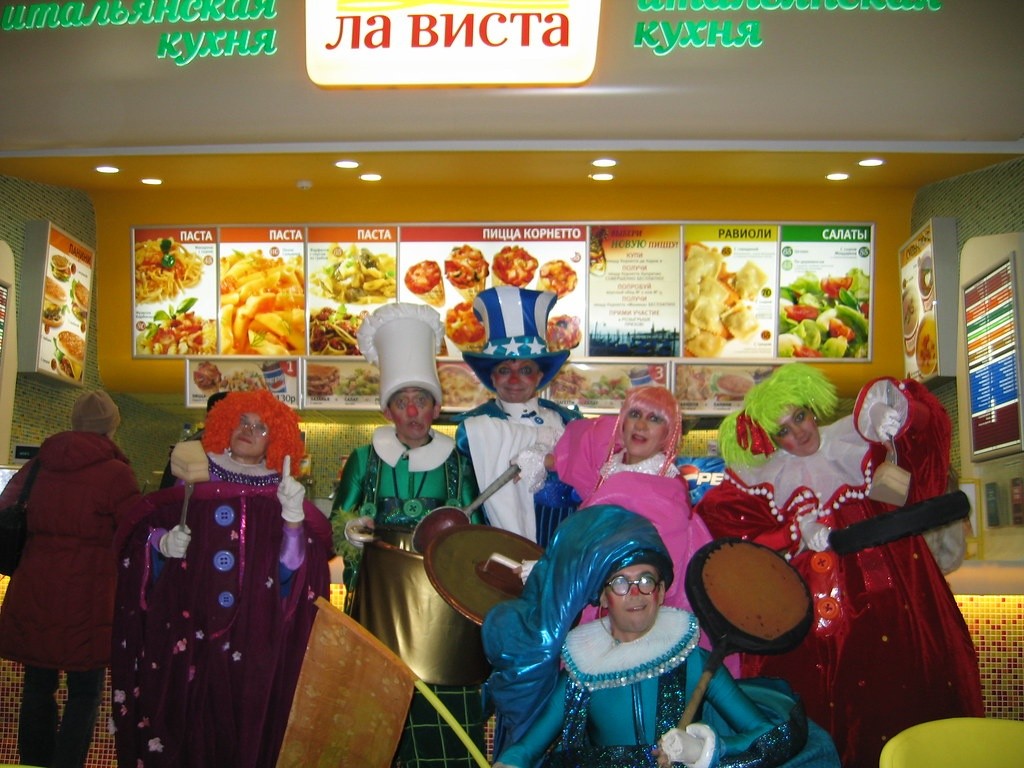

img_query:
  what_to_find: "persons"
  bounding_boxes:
[490,540,841,768]
[0,389,143,768]
[324,368,486,688]
[455,340,588,554]
[577,380,742,679]
[695,358,989,768]
[111,386,333,768]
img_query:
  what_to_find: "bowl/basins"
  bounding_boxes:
[715,369,756,397]
[436,365,477,384]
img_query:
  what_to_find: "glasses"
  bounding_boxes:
[236,420,269,440]
[604,575,660,596]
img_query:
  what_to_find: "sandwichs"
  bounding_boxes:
[306,363,340,397]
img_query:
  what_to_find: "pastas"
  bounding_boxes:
[134,236,207,302]
[136,313,217,357]
[312,241,395,305]
[218,251,307,356]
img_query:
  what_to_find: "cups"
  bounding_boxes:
[902,283,920,357]
[915,311,937,381]
[917,251,934,311]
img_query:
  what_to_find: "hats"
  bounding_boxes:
[71,390,120,434]
[461,286,571,394]
[356,302,446,409]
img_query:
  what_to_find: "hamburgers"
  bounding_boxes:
[41,255,93,383]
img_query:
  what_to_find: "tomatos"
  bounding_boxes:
[819,277,853,294]
[861,303,869,318]
[792,345,821,357]
[829,317,856,341]
[784,305,820,321]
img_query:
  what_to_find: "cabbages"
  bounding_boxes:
[778,269,870,359]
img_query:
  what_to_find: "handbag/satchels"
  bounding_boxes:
[0,458,40,576]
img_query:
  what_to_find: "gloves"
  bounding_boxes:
[869,402,902,444]
[159,525,192,560]
[276,455,305,523]
[798,513,831,552]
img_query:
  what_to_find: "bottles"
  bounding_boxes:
[179,423,194,442]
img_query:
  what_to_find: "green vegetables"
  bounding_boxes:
[580,374,632,400]
[145,296,199,340]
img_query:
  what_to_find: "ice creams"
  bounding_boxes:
[405,245,581,353]
